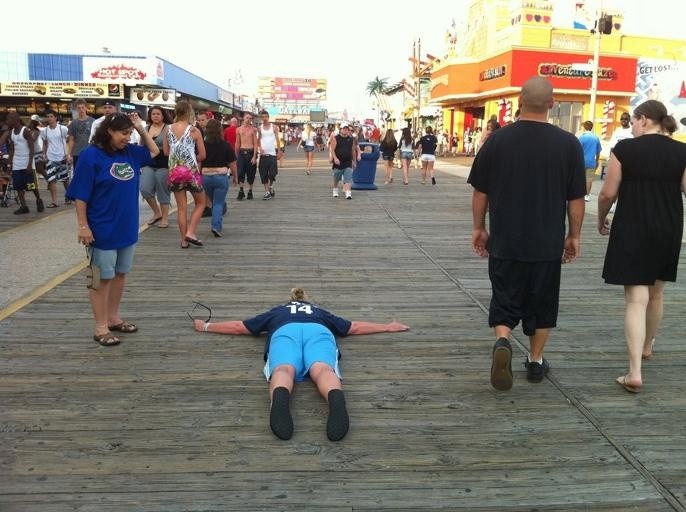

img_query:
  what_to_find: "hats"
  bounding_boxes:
[103,101,115,106]
[340,123,348,128]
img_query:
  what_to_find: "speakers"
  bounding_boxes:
[603,15,612,34]
[599,18,606,32]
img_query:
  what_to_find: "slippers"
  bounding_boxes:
[109,320,138,333]
[643,337,657,360]
[617,375,640,393]
[181,235,203,249]
[94,332,120,346]
[148,216,169,228]
[431,177,436,186]
[46,198,71,208]
[270,387,293,440]
[324,389,349,442]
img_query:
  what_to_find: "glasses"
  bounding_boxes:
[188,300,211,322]
[110,114,128,121]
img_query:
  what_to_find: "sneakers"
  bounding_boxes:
[212,228,222,236]
[489,337,514,390]
[584,195,590,201]
[237,189,252,199]
[346,191,351,199]
[14,199,44,214]
[332,188,338,197]
[525,355,549,383]
[263,185,275,200]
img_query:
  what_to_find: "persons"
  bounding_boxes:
[466,77,587,391]
[437,109,520,158]
[193,286,410,440]
[596,99,686,392]
[63,112,161,346]
[0,100,284,247]
[578,112,635,212]
[284,123,438,199]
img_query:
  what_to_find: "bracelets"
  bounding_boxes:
[203,323,211,331]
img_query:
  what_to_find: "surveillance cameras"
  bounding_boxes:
[590,28,596,34]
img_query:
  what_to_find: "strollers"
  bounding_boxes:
[0,155,22,207]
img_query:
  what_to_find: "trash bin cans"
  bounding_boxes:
[351,142,381,190]
